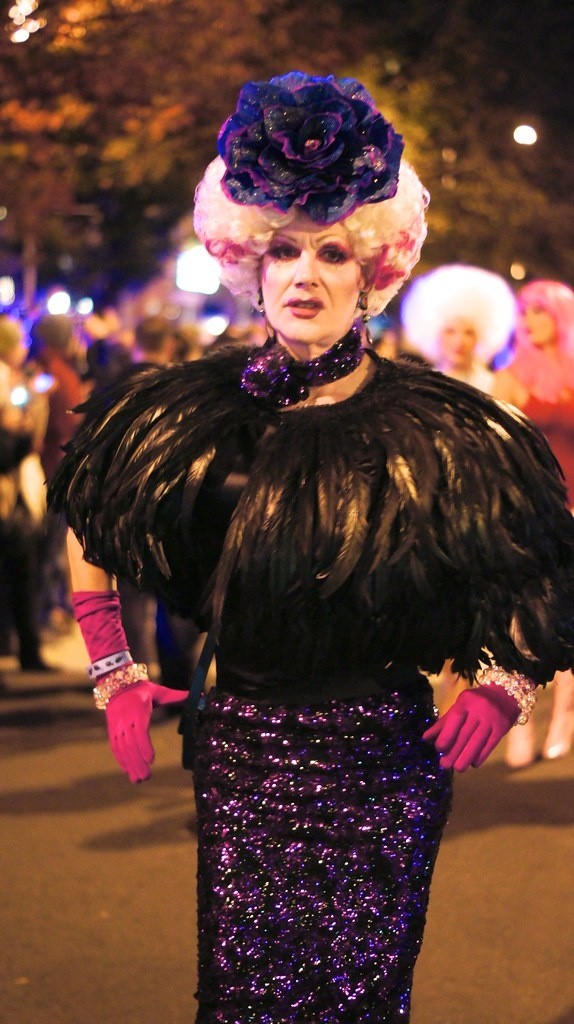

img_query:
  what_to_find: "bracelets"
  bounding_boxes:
[477,663,539,726]
[93,662,148,709]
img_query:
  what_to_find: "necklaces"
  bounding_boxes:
[242,325,363,410]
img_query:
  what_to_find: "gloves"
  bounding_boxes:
[421,681,521,773]
[70,590,190,784]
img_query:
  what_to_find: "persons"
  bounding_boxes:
[0,302,275,711]
[361,264,574,768]
[43,69,574,1024]
